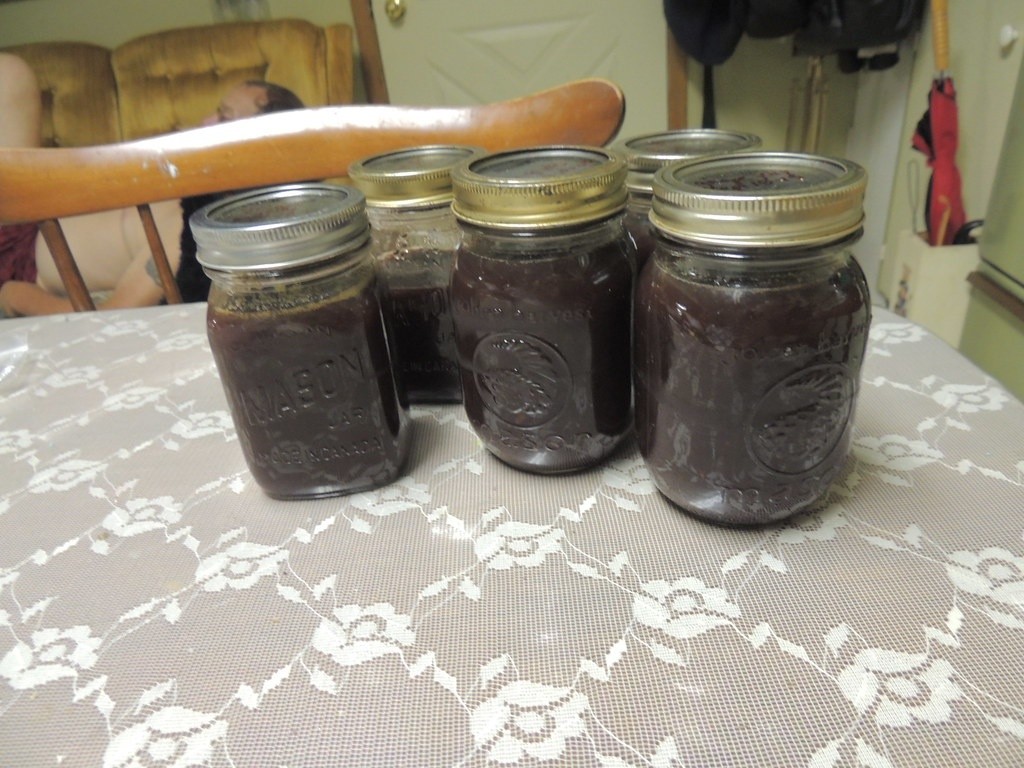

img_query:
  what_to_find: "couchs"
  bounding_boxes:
[0,19,371,187]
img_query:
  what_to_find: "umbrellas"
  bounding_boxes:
[911,0,964,245]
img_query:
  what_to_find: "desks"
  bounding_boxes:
[2,301,1024,768]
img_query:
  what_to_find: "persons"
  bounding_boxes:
[0,51,308,316]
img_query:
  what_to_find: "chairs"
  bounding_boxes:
[0,80,632,313]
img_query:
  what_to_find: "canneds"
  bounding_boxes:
[188,129,873,529]
